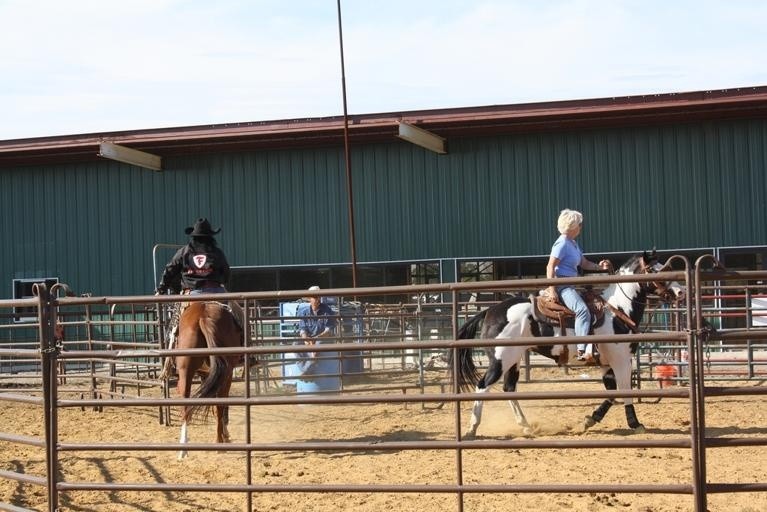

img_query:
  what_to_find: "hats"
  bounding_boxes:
[309,286,320,291]
[183,217,223,236]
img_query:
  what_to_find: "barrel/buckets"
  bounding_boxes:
[292,341,341,393]
[657,365,674,386]
[341,351,364,373]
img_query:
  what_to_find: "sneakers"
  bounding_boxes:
[576,351,600,362]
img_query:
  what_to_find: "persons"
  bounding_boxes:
[291,284,338,383]
[544,208,610,362]
[154,219,262,377]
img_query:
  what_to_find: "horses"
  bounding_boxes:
[445,245,686,441]
[173,300,244,462]
